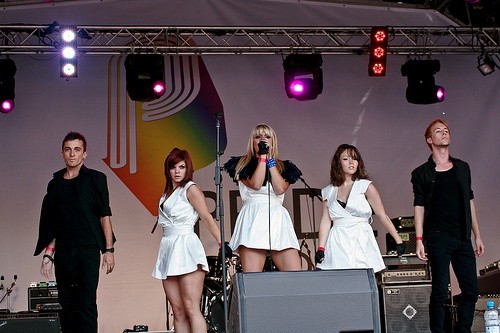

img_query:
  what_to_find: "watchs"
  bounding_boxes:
[106,247,114,252]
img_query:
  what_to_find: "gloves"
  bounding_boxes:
[315,247,325,263]
[396,244,406,256]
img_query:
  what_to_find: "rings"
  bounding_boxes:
[43,254,53,264]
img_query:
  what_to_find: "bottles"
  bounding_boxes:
[483,301,500,333]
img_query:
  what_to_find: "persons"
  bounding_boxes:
[314,143,404,275]
[225,123,302,272]
[152,148,234,333]
[411,119,484,333]
[33,133,117,333]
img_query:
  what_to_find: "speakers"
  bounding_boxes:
[379,283,432,333]
[228,268,381,333]
[0,313,61,333]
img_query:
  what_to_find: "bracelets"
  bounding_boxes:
[267,159,276,168]
[47,247,54,253]
[219,243,221,247]
[258,158,266,163]
[415,237,422,240]
[318,247,324,251]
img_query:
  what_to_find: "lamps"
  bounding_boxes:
[477,51,495,76]
[401,57,446,105]
[282,53,324,101]
[60,26,78,78]
[125,54,166,102]
[369,26,389,78]
[0,58,16,112]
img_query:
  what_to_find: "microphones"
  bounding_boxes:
[265,143,270,149]
[13,275,17,284]
[1,276,4,288]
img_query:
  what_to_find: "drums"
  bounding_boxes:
[208,289,232,333]
[206,255,238,283]
[236,254,271,273]
